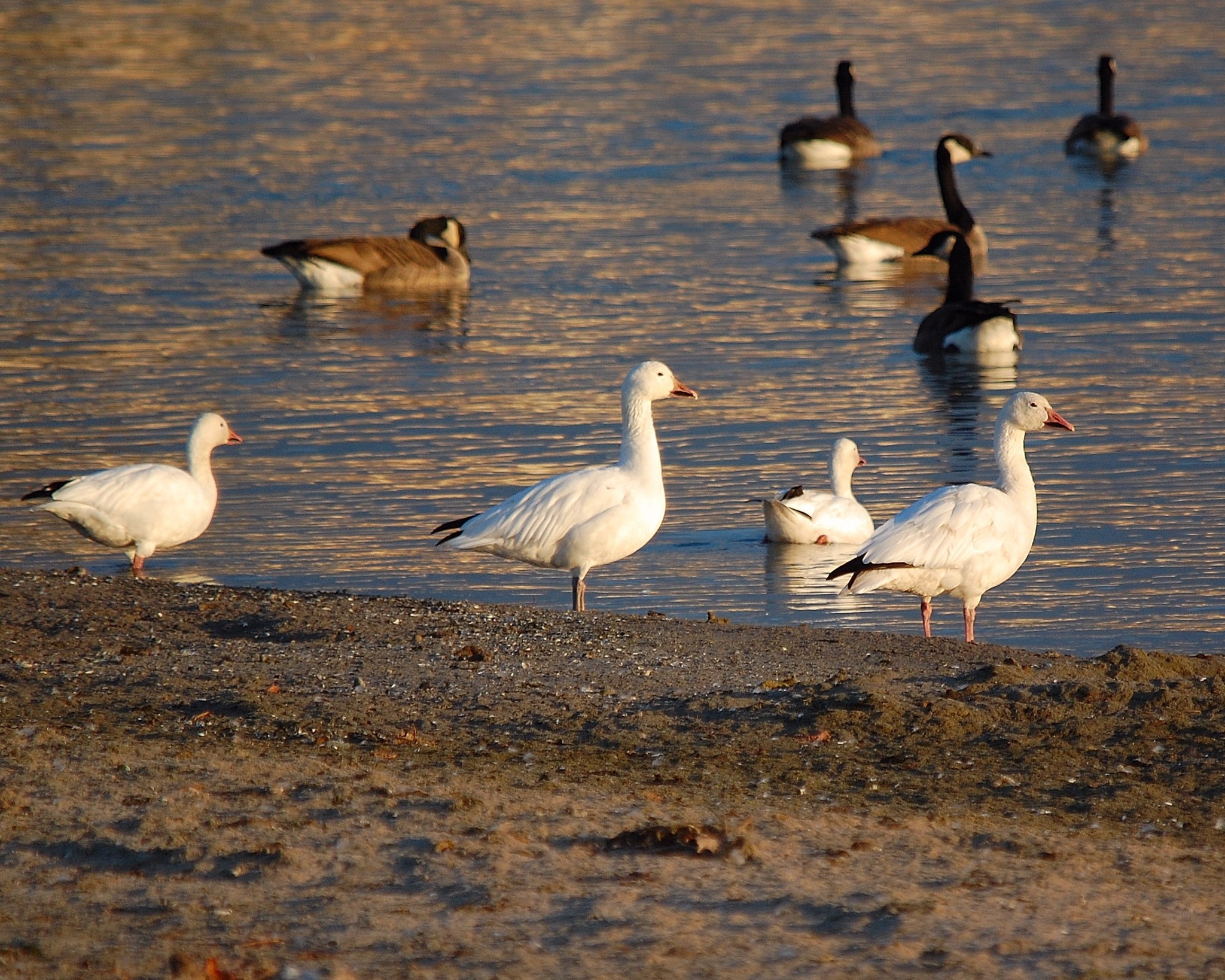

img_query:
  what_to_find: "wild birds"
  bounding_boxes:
[259,216,474,301]
[780,59,1025,364]
[22,411,243,583]
[743,392,1075,644]
[1064,51,1149,163]
[428,359,698,612]
